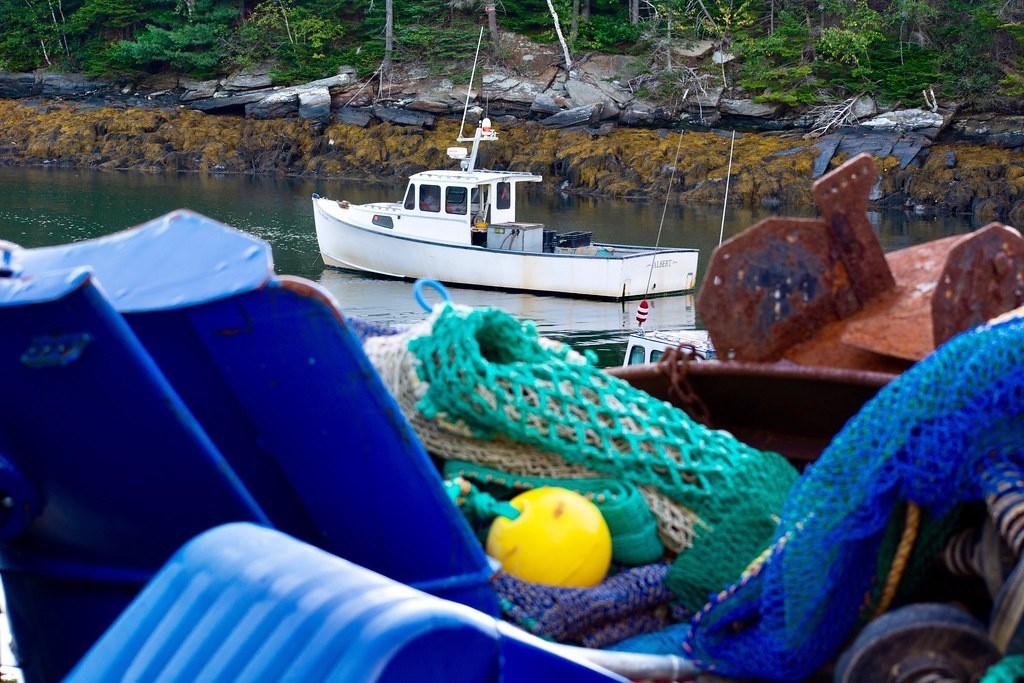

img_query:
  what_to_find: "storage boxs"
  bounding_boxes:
[542,230,593,253]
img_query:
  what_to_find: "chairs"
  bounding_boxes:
[421,202,429,210]
[436,200,452,212]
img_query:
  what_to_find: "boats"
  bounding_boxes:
[310,26,700,301]
[622,126,734,366]
[314,266,699,347]
